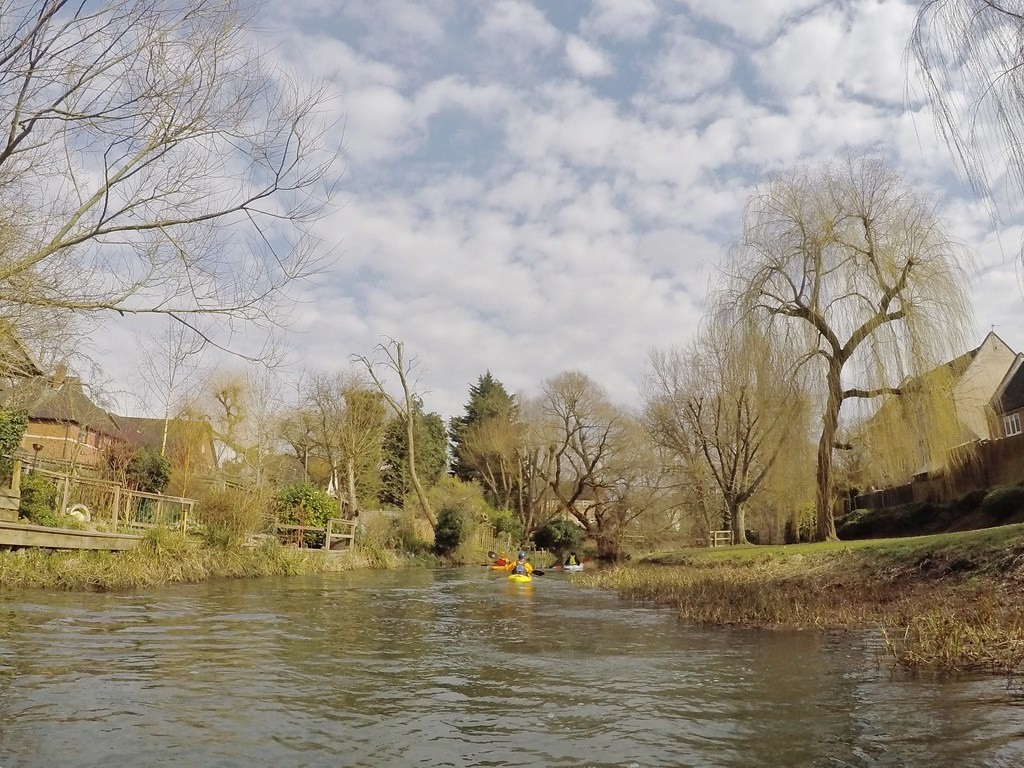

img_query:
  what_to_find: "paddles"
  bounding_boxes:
[488,551,546,576]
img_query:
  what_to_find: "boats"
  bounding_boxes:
[490,565,507,571]
[507,573,532,583]
[563,563,584,573]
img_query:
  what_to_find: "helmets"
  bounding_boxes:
[571,550,576,555]
[518,552,526,557]
[500,551,505,555]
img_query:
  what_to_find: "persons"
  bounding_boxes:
[505,552,533,578]
[565,551,581,566]
